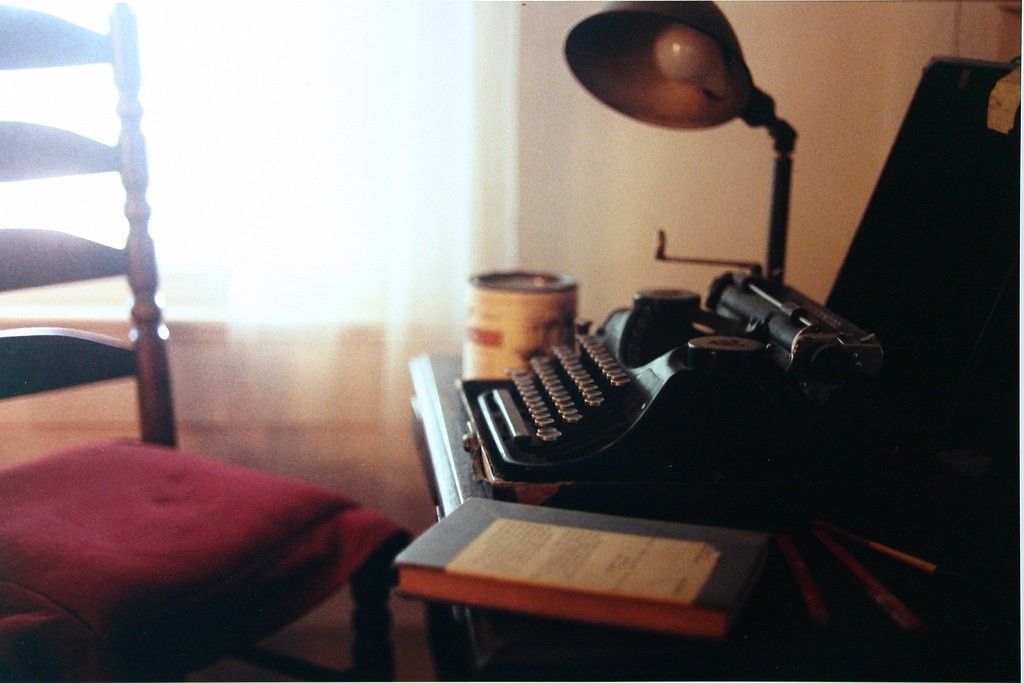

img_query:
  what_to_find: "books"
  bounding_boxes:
[390,495,774,640]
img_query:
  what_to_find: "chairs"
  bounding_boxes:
[0,1,416,682]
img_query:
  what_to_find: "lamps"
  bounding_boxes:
[564,0,802,278]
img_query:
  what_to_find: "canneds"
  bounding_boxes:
[461,270,576,381]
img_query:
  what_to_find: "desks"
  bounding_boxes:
[406,346,1020,682]
